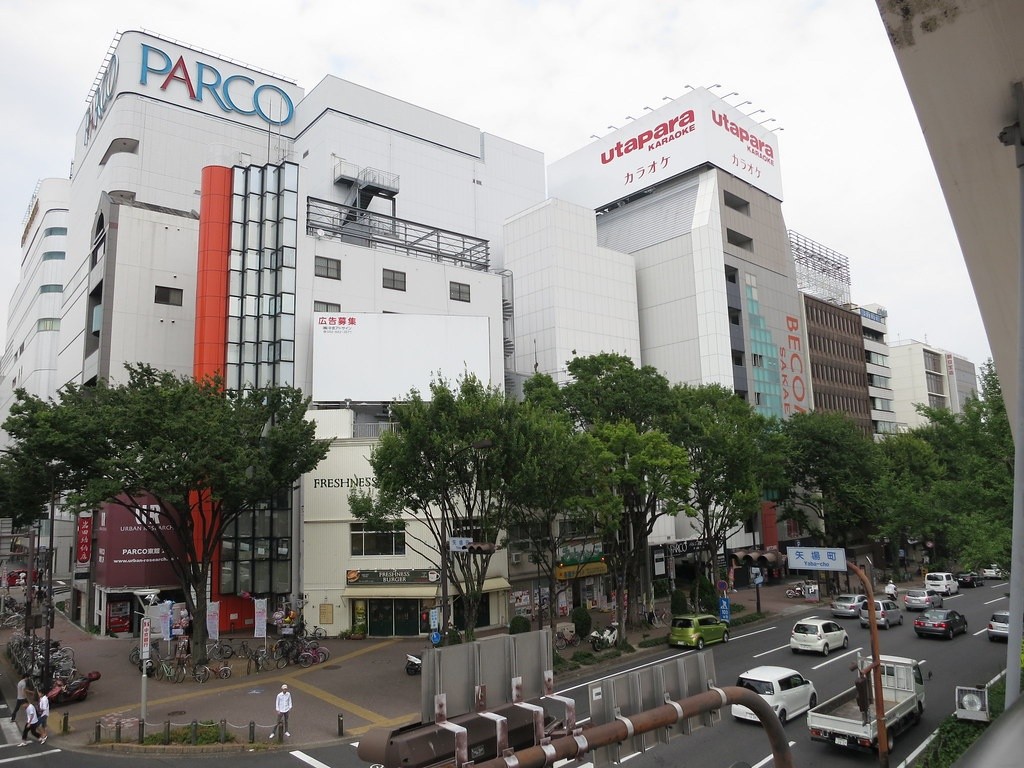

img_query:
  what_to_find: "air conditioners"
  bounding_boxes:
[511,553,522,564]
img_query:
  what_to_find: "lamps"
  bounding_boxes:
[746,109,766,118]
[721,92,739,100]
[707,83,721,91]
[643,106,653,112]
[591,134,601,139]
[661,95,674,102]
[771,126,784,133]
[734,100,752,109]
[625,116,636,121]
[685,84,698,92]
[759,118,776,125]
[608,124,618,130]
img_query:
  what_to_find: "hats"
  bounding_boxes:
[889,580,893,584]
[282,685,288,690]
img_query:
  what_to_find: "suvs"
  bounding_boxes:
[904,588,943,612]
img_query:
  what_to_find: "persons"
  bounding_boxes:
[19,569,48,612]
[10,674,49,747]
[269,684,292,738]
[888,579,898,598]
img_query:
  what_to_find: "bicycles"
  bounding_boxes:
[0,597,83,697]
[650,606,673,629]
[128,620,331,685]
[556,626,580,650]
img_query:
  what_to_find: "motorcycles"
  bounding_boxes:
[590,622,619,652]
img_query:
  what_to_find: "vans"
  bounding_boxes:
[859,600,904,630]
[925,572,959,597]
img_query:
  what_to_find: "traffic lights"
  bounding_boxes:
[462,542,496,555]
[730,550,784,569]
[422,611,430,623]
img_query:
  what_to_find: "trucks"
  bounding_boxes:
[806,654,933,756]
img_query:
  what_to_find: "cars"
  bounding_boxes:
[914,607,967,641]
[954,570,984,588]
[0,568,38,587]
[830,593,868,618]
[988,611,1009,642]
[732,665,817,727]
[982,564,1001,580]
[790,619,849,657]
[668,614,731,651]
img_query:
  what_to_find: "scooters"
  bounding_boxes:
[785,578,814,599]
[403,653,422,676]
[886,583,900,602]
[44,670,101,710]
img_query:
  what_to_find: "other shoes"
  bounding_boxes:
[10,719,17,722]
[17,743,27,747]
[41,736,47,744]
[285,732,290,736]
[269,733,274,738]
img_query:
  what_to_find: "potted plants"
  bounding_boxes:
[351,623,367,640]
